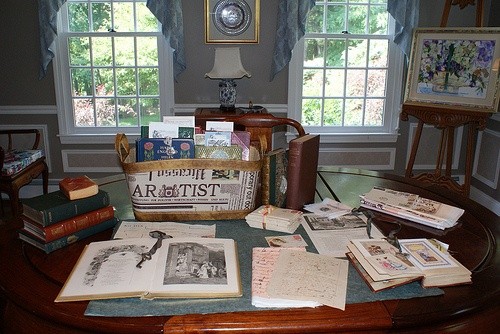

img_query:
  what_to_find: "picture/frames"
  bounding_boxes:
[403,24,500,114]
[203,0,260,46]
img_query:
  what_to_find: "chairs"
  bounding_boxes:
[0,128,49,220]
[219,114,306,153]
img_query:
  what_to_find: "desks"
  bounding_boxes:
[194,106,249,131]
[0,165,500,334]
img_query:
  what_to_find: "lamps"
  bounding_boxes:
[204,47,252,112]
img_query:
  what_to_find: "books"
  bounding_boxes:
[345,238,474,293]
[18,175,116,254]
[135,126,250,161]
[53,238,243,300]
